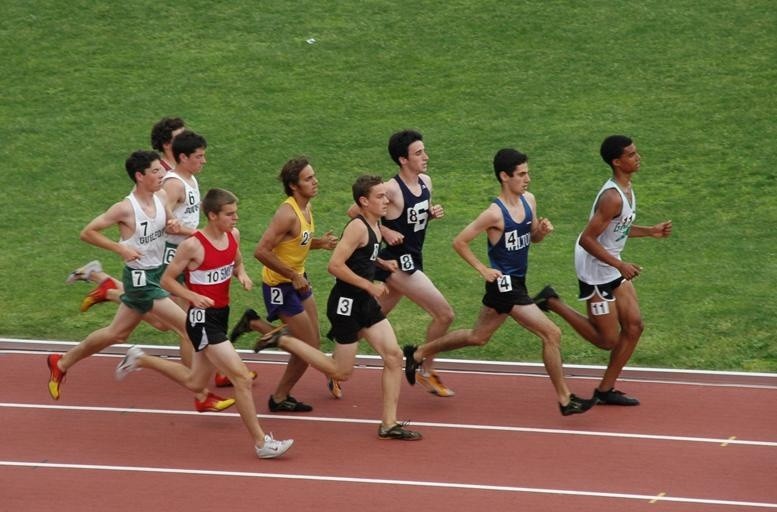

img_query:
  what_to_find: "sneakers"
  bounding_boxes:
[269,392,314,411]
[323,371,343,400]
[404,342,422,384]
[593,388,639,406]
[416,370,455,399]
[229,308,262,343]
[193,392,237,412]
[64,259,102,283]
[114,345,145,381]
[254,322,292,355]
[561,393,592,416]
[254,432,294,458]
[531,284,558,310]
[213,368,257,388]
[376,423,423,441]
[81,276,117,313]
[46,351,66,401]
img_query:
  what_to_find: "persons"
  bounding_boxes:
[254,173,422,440]
[66,117,186,288]
[532,135,672,406]
[115,188,294,459]
[403,148,598,417]
[327,129,454,398]
[80,132,257,387]
[46,150,236,412]
[230,158,337,411]
